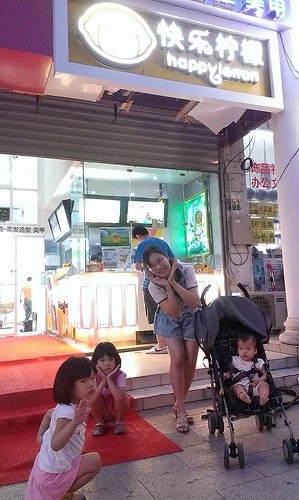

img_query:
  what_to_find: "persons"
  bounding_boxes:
[223,334,269,406]
[132,226,176,354]
[87,341,127,436]
[143,245,203,433]
[25,355,102,500]
[20,276,33,321]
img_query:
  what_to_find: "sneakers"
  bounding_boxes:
[146,344,168,354]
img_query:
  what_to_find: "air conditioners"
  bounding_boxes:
[232,290,287,331]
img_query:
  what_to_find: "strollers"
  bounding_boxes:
[194,283,297,469]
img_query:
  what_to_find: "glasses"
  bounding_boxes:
[149,255,165,271]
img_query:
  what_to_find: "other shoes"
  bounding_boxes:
[114,422,124,434]
[91,424,106,435]
[61,493,86,500]
[176,412,189,432]
[173,405,194,424]
